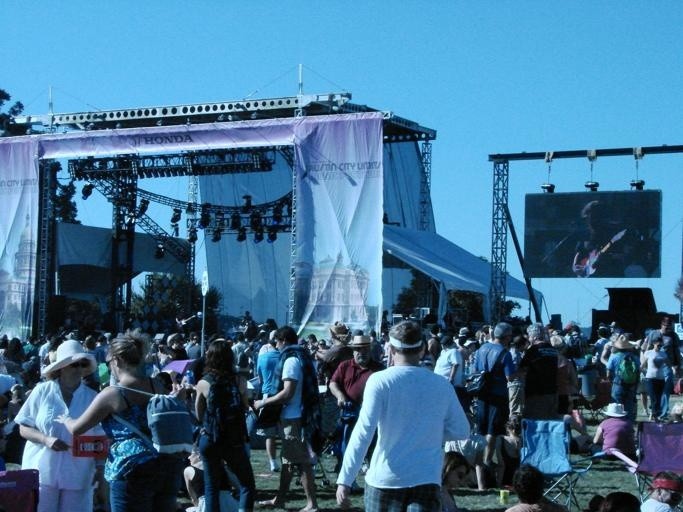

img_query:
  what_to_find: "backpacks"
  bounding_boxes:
[110,384,192,455]
[200,374,251,447]
[615,352,639,385]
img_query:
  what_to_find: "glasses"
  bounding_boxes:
[454,469,466,481]
[70,361,90,369]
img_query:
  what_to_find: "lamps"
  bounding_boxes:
[585,181,599,191]
[541,184,555,192]
[631,180,645,190]
[25,112,294,259]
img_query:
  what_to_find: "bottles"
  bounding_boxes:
[186,368,194,384]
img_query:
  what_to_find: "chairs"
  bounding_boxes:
[520,419,593,512]
[628,422,683,504]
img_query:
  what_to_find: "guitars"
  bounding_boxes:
[572,228,627,278]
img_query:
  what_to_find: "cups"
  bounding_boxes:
[499,489,509,504]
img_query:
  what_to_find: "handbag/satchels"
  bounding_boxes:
[464,370,489,396]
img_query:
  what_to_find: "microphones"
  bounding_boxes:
[571,214,588,227]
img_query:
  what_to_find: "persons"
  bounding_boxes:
[0,309,683,512]
[571,200,636,277]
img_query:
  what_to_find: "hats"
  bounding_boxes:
[43,340,98,378]
[600,403,629,418]
[347,336,378,349]
[608,335,635,350]
[458,327,468,337]
[439,336,451,345]
